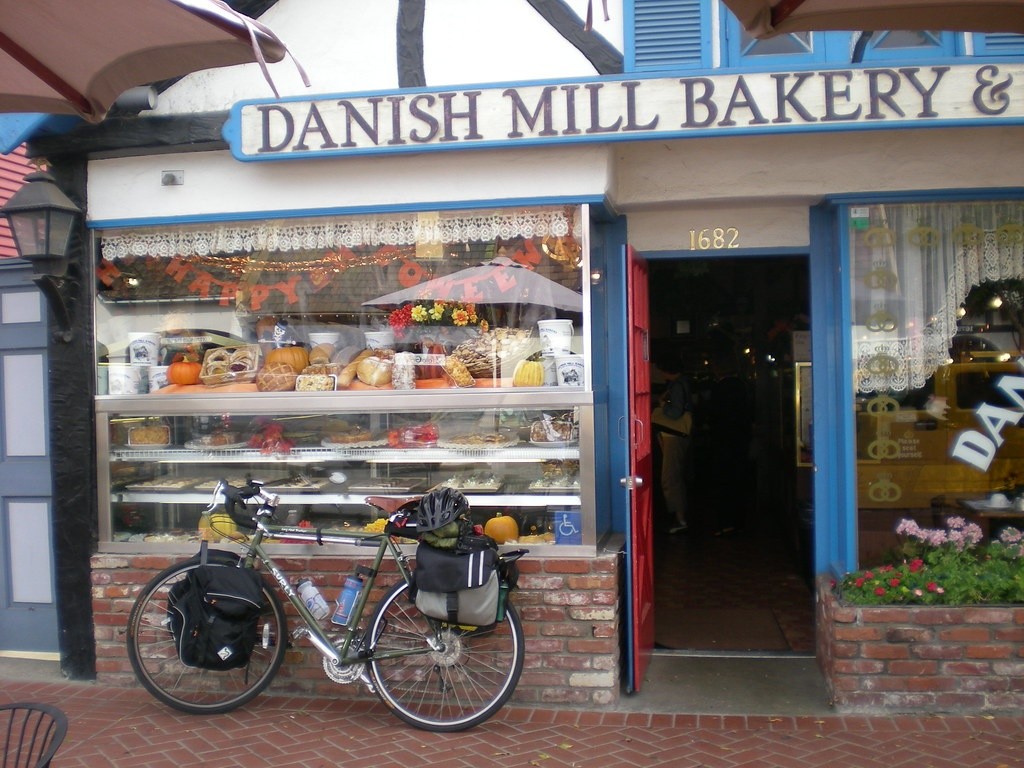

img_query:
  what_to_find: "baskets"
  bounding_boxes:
[199,344,260,387]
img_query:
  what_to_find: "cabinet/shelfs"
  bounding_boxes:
[95,382,612,558]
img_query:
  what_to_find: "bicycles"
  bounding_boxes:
[126,471,525,733]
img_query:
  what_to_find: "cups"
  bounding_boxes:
[363,332,394,350]
[308,332,341,352]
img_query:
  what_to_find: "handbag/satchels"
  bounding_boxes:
[168,564,266,670]
[406,542,499,627]
[650,381,695,438]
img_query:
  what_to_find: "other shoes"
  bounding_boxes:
[666,523,688,534]
[712,528,744,538]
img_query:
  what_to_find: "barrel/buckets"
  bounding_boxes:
[146,366,171,393]
[127,332,160,367]
[535,354,559,386]
[537,318,575,356]
[107,363,142,396]
[554,352,584,386]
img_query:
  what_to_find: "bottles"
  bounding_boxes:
[331,572,362,626]
[295,577,329,620]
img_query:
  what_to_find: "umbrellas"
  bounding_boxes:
[0,0,286,122]
[357,256,585,435]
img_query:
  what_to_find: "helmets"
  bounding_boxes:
[417,487,469,533]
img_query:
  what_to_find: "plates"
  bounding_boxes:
[529,440,580,449]
[124,443,172,449]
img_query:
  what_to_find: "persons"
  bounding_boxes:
[707,350,752,536]
[652,380,695,534]
